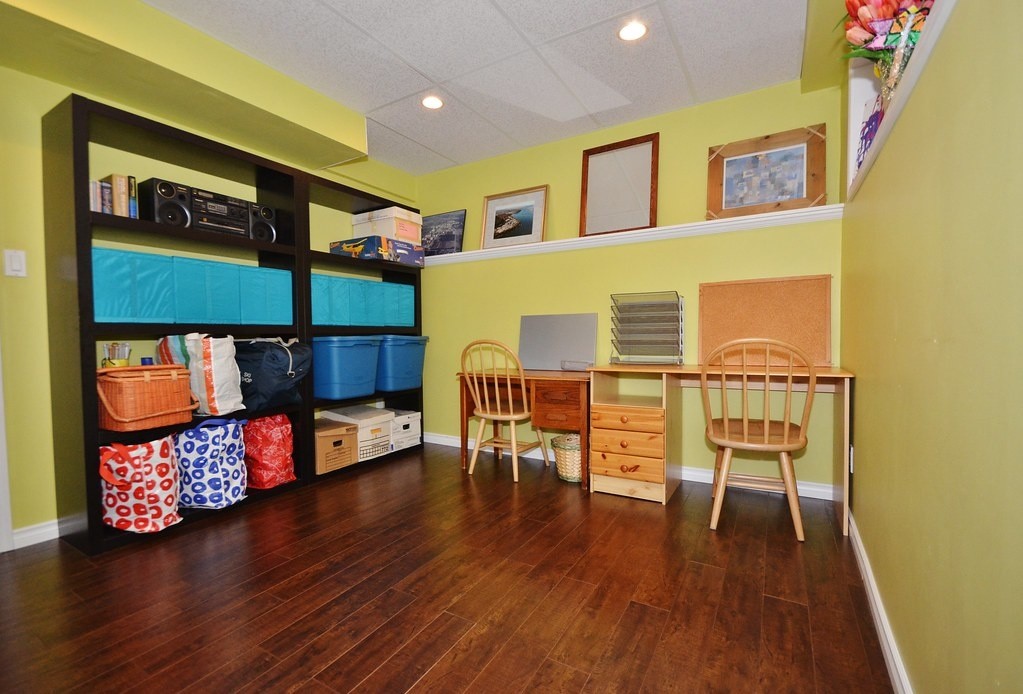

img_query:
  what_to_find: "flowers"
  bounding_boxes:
[832,0,936,67]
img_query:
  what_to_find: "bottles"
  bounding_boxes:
[141,357,153,366]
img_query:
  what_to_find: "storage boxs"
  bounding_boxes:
[321,404,395,463]
[383,282,414,327]
[374,335,430,391]
[383,408,422,453]
[329,235,425,269]
[239,264,293,325]
[172,256,241,324]
[91,246,176,324]
[312,273,350,326]
[312,335,383,401]
[347,277,385,326]
[315,417,358,475]
[351,206,423,246]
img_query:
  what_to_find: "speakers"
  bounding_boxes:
[246,202,276,244]
[138,177,193,228]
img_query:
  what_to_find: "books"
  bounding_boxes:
[88,173,140,220]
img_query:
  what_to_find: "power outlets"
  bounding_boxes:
[849,443,854,474]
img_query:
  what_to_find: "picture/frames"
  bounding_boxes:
[706,122,827,220]
[422,208,467,256]
[479,183,551,249]
[578,132,660,237]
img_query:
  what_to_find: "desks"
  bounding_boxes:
[455,367,591,490]
[587,364,856,536]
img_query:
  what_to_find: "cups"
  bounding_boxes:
[102,358,128,368]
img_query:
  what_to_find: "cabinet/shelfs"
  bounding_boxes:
[41,92,425,557]
[610,291,685,366]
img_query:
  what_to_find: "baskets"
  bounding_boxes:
[551,434,582,483]
[97,364,200,432]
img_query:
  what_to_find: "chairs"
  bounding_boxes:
[460,338,550,483]
[700,337,818,542]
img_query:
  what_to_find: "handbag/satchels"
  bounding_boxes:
[175,419,250,511]
[243,414,298,490]
[233,337,312,413]
[155,333,247,417]
[100,434,185,535]
